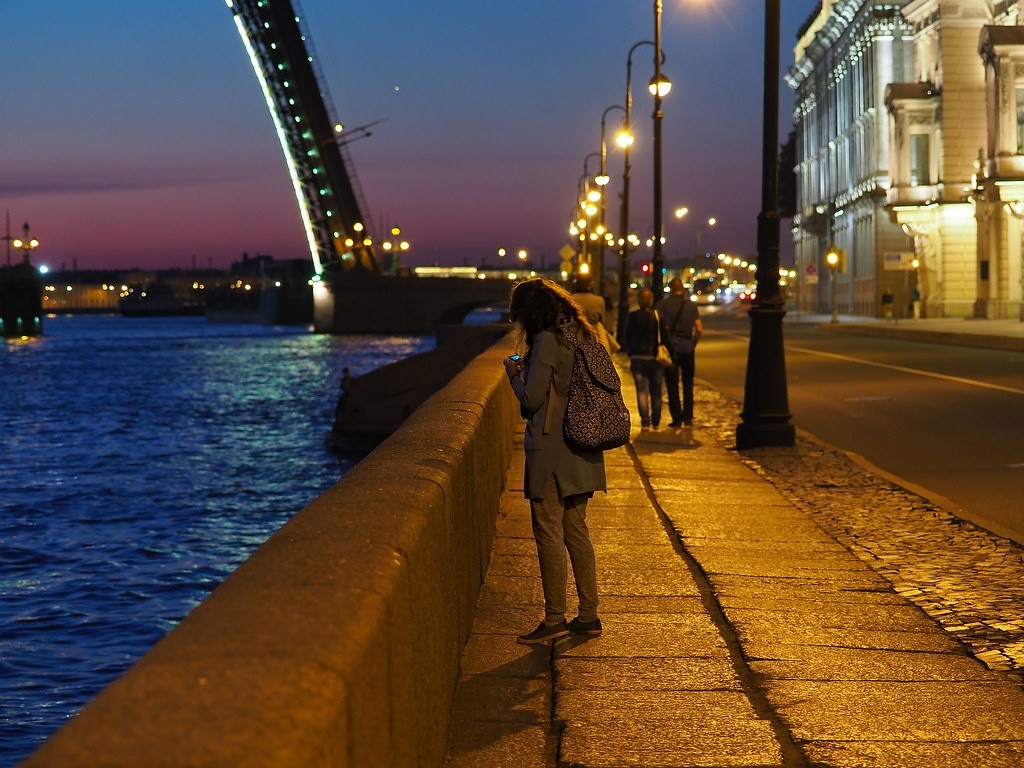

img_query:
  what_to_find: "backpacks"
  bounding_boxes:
[555,320,632,450]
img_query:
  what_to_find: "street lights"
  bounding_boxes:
[597,105,635,309]
[615,41,658,343]
[568,153,610,294]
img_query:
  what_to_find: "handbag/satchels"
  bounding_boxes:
[656,343,671,369]
[660,338,672,353]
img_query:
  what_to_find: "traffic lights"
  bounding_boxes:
[827,253,839,265]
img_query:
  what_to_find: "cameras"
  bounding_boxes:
[508,354,520,363]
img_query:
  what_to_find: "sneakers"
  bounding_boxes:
[567,618,602,635]
[517,620,568,643]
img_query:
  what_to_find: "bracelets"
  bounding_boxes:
[510,374,520,385]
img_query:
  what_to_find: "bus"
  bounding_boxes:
[692,273,717,306]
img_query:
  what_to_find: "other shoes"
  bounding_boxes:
[684,420,693,426]
[652,424,658,429]
[641,426,649,431]
[668,422,681,427]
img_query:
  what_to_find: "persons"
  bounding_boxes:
[661,279,703,429]
[624,288,664,432]
[912,285,921,321]
[569,276,606,325]
[602,297,615,337]
[882,287,894,322]
[503,277,608,644]
